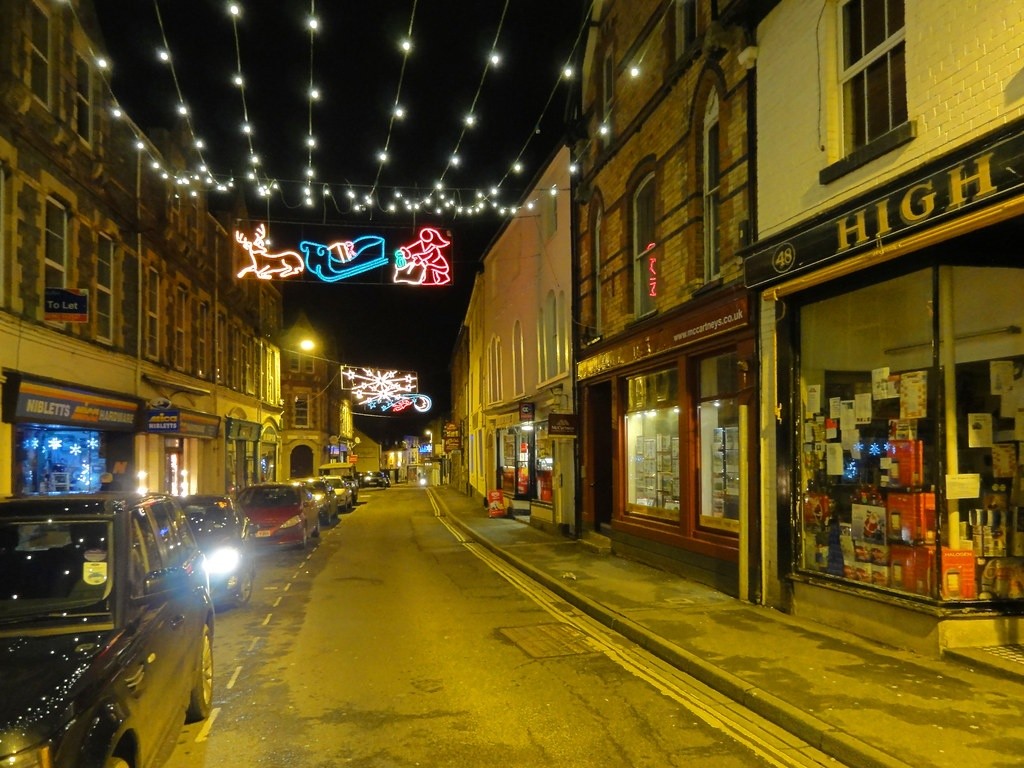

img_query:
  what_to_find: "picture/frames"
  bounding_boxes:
[634,435,680,500]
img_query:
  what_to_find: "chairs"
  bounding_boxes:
[45,524,107,598]
[0,525,24,564]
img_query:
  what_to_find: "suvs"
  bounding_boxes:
[0,490,216,768]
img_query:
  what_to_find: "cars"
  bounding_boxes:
[341,476,359,502]
[325,477,353,511]
[235,482,320,550]
[171,494,256,608]
[290,478,338,526]
[363,471,390,487]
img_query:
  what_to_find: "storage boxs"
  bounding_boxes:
[804,437,1024,601]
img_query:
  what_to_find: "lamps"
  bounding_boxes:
[883,326,1022,355]
